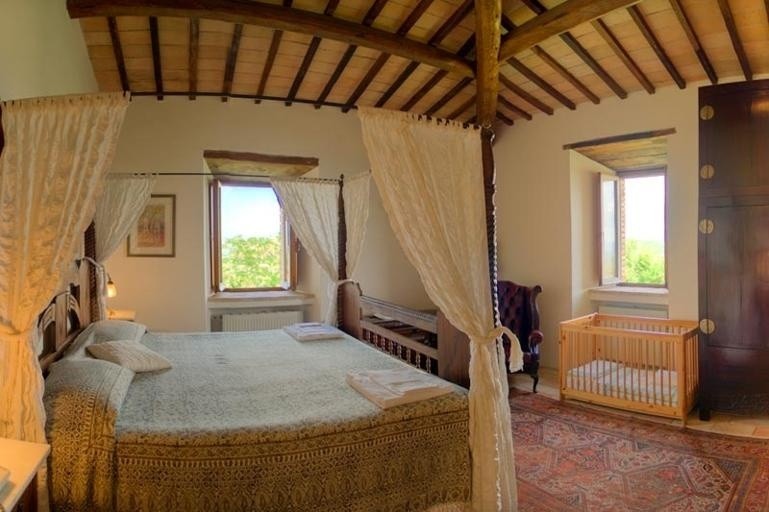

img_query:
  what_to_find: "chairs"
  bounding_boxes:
[496,280,544,392]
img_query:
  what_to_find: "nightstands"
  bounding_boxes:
[0,439,51,512]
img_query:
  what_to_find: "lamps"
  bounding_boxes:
[477,111,497,145]
[75,256,115,298]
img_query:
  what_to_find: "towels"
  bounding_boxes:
[347,366,455,413]
[281,321,343,341]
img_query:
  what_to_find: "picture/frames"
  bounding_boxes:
[127,194,176,258]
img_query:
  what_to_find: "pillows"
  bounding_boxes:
[85,338,174,373]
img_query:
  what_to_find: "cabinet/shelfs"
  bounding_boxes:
[697,86,769,423]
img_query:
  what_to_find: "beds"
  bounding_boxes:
[560,311,699,426]
[39,279,474,512]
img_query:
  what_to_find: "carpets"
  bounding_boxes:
[507,386,768,512]
